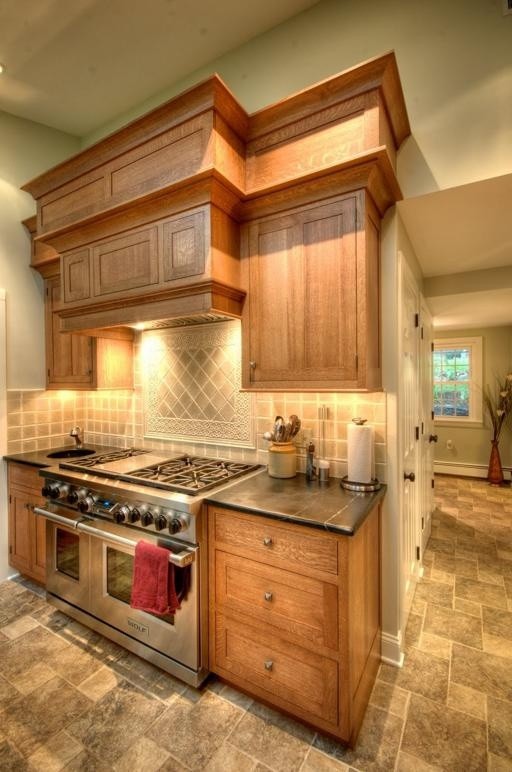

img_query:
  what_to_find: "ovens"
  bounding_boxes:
[33,502,208,688]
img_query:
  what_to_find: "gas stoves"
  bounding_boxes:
[38,447,261,544]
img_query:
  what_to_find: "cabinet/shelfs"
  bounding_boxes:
[42,274,93,384]
[247,196,359,383]
[398,251,438,632]
[203,505,387,750]
[7,462,46,588]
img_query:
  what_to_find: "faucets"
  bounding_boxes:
[70,425,82,448]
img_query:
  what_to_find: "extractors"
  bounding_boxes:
[52,278,246,340]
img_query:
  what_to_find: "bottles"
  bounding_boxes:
[267,441,297,479]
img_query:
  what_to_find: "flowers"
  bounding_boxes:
[478,367,512,440]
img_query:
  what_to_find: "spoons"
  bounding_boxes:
[263,414,301,442]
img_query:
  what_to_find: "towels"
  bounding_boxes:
[130,541,181,617]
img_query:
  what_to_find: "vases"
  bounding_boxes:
[488,440,503,487]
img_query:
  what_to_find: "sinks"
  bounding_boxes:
[45,446,97,459]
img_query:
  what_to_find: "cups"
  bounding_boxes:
[319,468,328,481]
